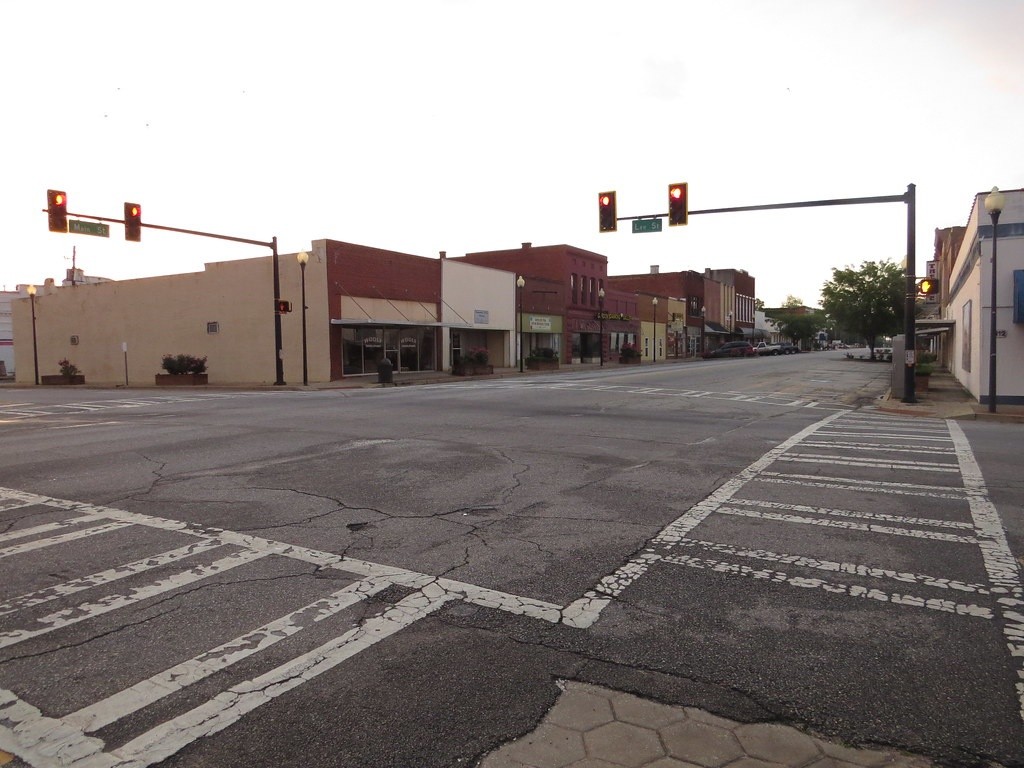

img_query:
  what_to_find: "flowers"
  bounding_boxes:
[454,344,489,369]
[620,342,642,361]
[699,350,721,359]
[745,345,760,354]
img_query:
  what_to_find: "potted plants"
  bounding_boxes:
[915,363,936,392]
[41,358,85,385]
[524,347,560,371]
[155,353,209,386]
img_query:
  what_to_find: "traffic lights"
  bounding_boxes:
[921,279,934,294]
[598,191,617,233]
[668,182,689,227]
[46,188,69,233]
[124,201,142,242]
[277,300,289,312]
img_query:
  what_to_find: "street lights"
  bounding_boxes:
[598,288,605,365]
[26,283,39,385]
[701,305,706,353]
[984,186,1006,413]
[652,297,658,362]
[729,310,733,341]
[517,276,525,372]
[297,247,310,387]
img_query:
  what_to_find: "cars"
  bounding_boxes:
[707,340,754,358]
[775,342,798,354]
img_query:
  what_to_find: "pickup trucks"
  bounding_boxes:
[752,342,782,356]
